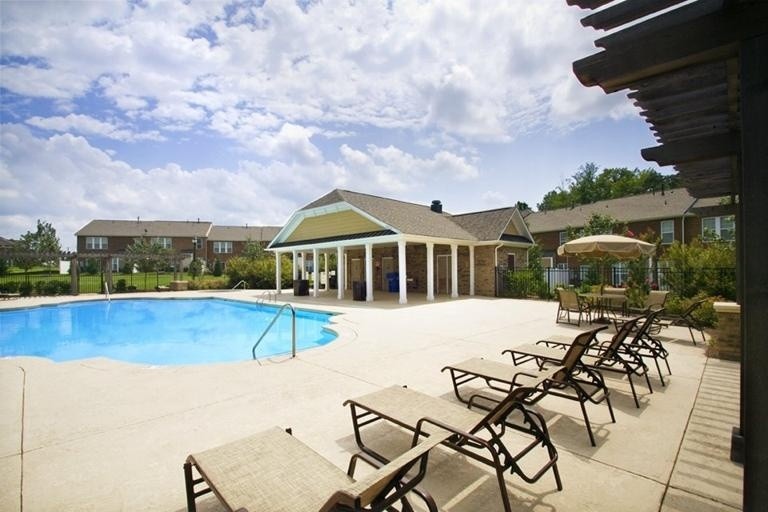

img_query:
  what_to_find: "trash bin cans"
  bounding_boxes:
[386,272,399,293]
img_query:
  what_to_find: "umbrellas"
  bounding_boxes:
[555,234,658,288]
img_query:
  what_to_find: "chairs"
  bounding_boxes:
[441,326,616,447]
[502,307,671,409]
[555,286,706,344]
[184,426,454,510]
[343,365,562,511]
